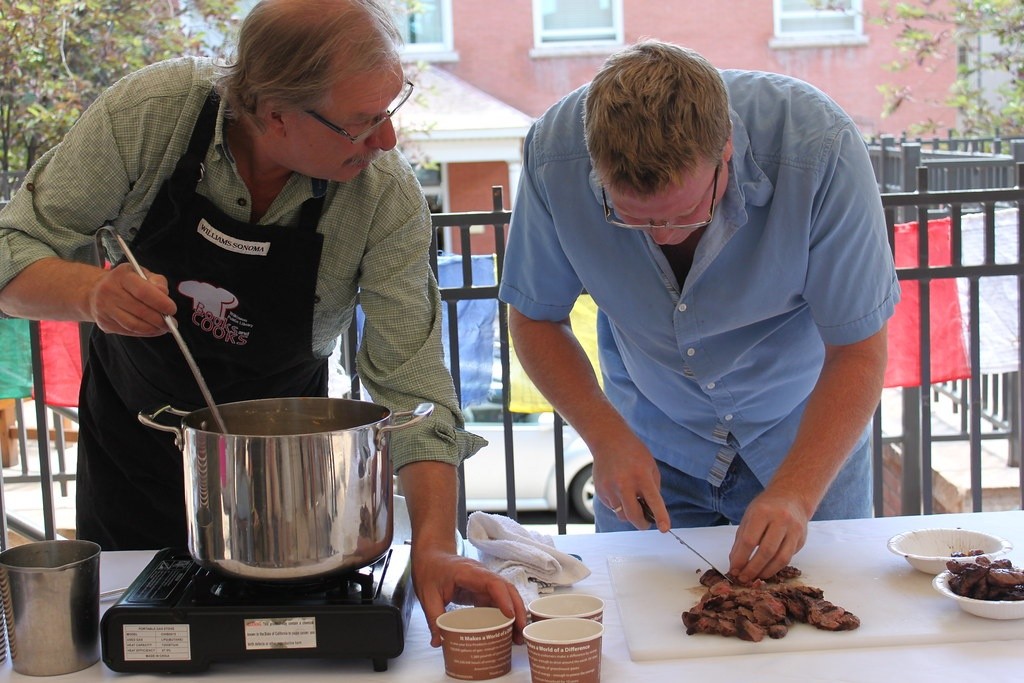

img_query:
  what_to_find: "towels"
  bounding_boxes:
[465,508,594,614]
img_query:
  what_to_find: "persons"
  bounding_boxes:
[0,1,527,647]
[498,38,901,584]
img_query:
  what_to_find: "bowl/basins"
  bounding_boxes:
[528,594,604,624]
[932,571,1024,619]
[888,528,1012,574]
[522,618,604,683]
[436,607,515,680]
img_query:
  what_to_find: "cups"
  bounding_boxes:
[0,540,104,676]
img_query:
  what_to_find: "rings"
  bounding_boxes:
[612,506,619,514]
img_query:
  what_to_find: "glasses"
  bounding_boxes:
[603,160,722,230]
[304,78,414,145]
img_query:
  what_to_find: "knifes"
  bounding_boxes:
[639,497,734,582]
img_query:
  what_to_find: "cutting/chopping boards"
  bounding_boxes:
[607,552,1024,661]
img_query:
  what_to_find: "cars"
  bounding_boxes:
[457,339,603,524]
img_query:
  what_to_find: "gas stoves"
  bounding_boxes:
[100,545,415,673]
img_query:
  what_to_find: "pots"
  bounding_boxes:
[137,399,434,581]
